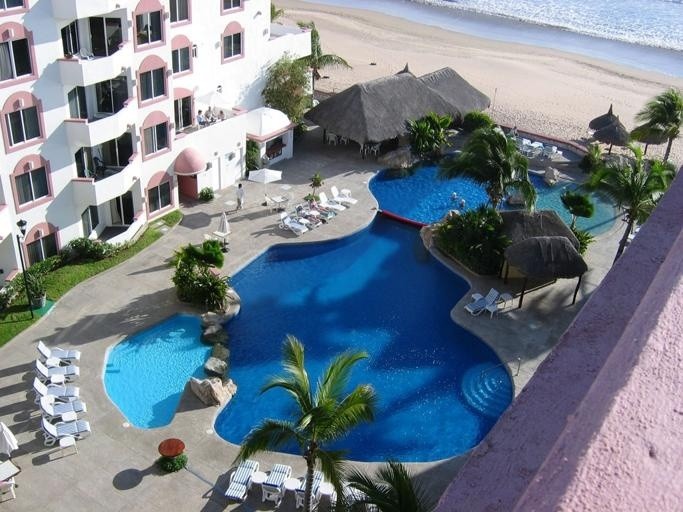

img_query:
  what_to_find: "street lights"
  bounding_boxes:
[16,218,33,320]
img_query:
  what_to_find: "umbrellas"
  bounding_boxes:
[248,168,283,194]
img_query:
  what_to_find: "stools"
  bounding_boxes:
[484,305,499,320]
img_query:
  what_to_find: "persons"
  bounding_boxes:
[310,197,332,216]
[235,183,244,210]
[196,105,226,125]
[297,206,320,224]
[510,126,520,138]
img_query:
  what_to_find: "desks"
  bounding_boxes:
[158,439,185,462]
[59,436,77,456]
[498,293,513,309]
[471,293,483,303]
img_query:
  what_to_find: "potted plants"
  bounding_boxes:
[24,270,46,308]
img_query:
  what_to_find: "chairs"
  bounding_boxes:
[0,460,16,498]
[506,132,563,160]
[31,340,91,446]
[280,185,357,237]
[464,288,499,320]
[224,459,379,512]
[325,130,381,159]
